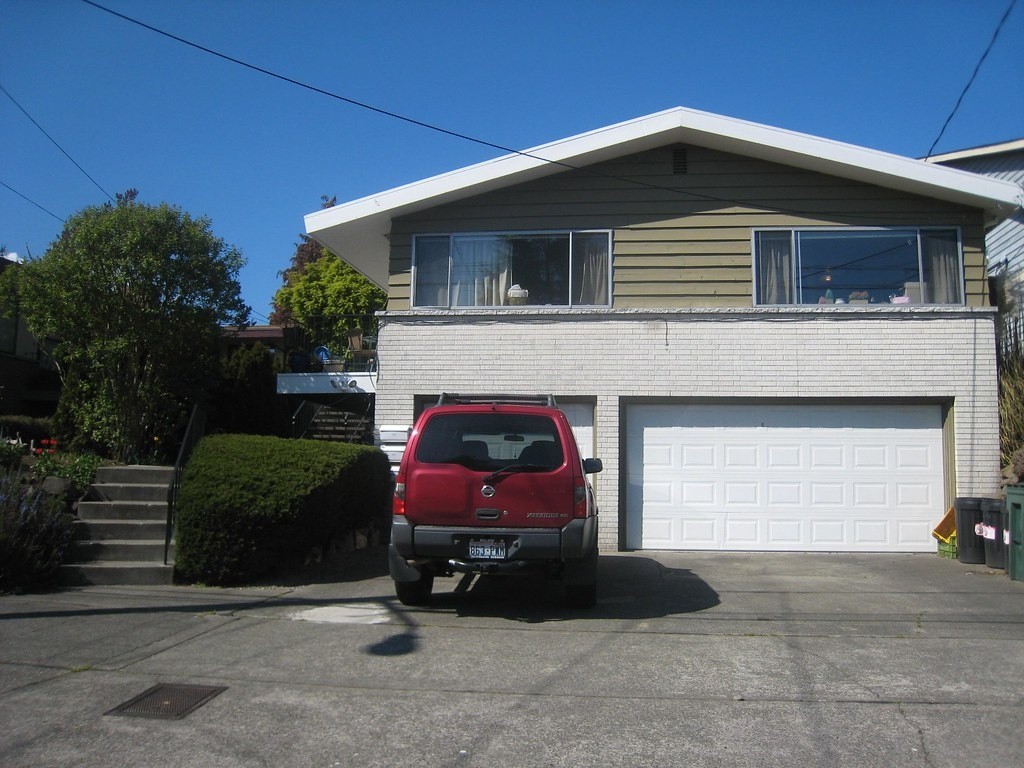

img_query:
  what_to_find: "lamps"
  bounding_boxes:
[822,267,832,281]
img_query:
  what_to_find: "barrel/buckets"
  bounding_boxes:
[954,497,985,564]
[905,281,927,304]
[981,498,1008,569]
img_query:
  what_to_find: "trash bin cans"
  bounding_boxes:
[953,497,1009,573]
[1007,482,1024,582]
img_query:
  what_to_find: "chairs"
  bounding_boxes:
[343,329,376,372]
[464,441,555,464]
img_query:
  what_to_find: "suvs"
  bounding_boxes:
[388,392,605,606]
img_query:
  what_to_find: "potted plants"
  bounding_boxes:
[825,289,833,304]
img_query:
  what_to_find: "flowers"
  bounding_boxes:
[848,291,869,300]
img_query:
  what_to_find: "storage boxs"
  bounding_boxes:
[940,537,958,559]
[933,506,957,544]
[322,360,346,371]
[849,300,868,304]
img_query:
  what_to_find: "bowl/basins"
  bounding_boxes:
[508,296,528,305]
